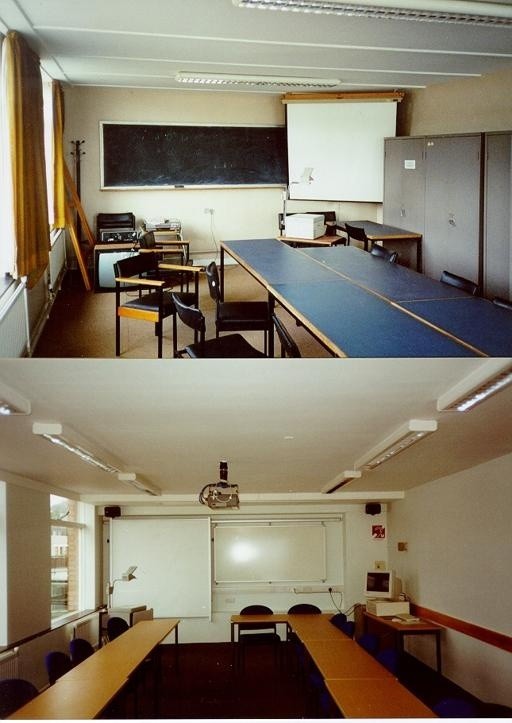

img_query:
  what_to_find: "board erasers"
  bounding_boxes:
[174,185,184,188]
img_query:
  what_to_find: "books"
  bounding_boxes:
[396,613,420,623]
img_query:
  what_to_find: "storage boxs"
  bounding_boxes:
[285,213,325,240]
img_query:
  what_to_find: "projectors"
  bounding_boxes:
[208,495,239,510]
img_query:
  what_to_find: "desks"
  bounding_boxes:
[361,611,443,679]
[277,234,347,247]
[217,237,512,357]
[326,219,423,273]
[230,613,438,717]
[0,618,181,719]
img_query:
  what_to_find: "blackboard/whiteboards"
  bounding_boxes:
[213,524,326,584]
[99,120,289,191]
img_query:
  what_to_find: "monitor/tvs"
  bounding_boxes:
[94,243,141,293]
[364,569,402,602]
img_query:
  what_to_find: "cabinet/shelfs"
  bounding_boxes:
[383,129,512,304]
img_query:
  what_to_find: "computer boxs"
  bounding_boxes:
[366,601,409,619]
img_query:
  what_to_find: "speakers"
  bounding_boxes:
[105,507,120,518]
[366,503,381,515]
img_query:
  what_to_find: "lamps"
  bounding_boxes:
[231,0,512,29]
[174,71,340,89]
[319,358,512,495]
[0,381,162,497]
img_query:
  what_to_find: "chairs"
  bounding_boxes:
[237,603,398,718]
[440,270,478,296]
[437,699,472,718]
[370,243,398,263]
[492,296,512,311]
[0,617,131,719]
[344,223,369,250]
[113,238,301,357]
